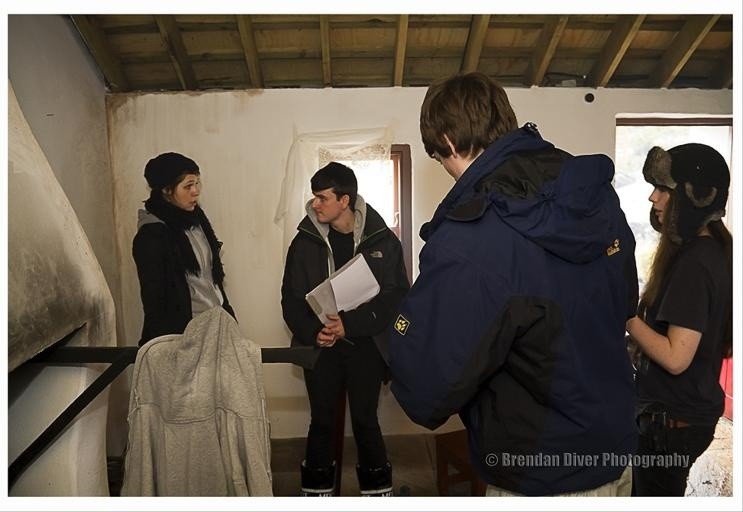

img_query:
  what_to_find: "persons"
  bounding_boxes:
[623,144,733,496]
[390,71,641,497]
[280,162,410,497]
[131,151,237,348]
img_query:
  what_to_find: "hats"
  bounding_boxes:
[643,142,730,245]
[143,151,200,188]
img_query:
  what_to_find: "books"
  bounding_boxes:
[301,250,380,328]
